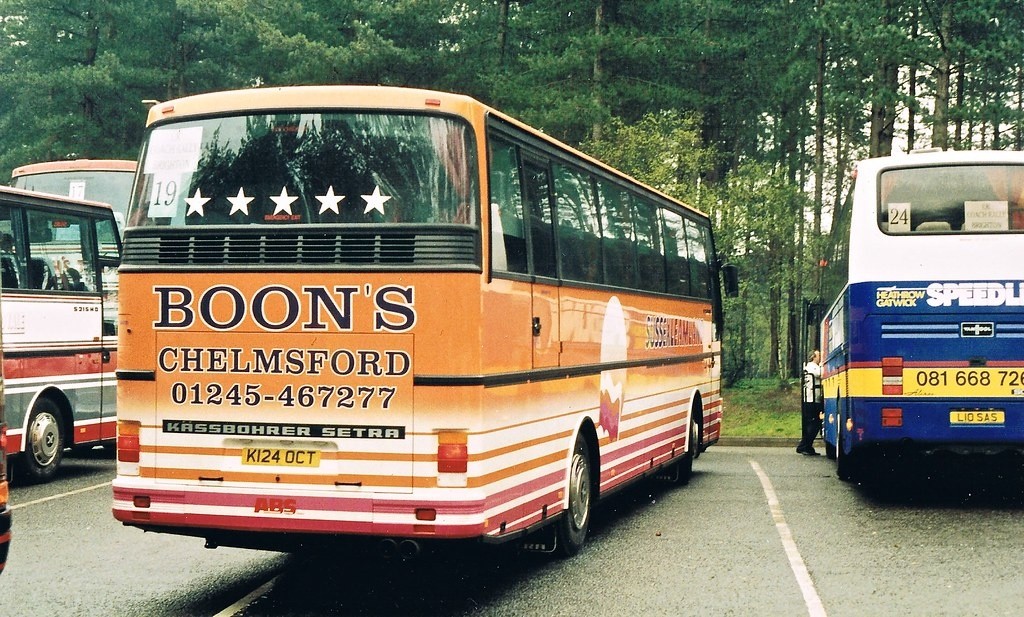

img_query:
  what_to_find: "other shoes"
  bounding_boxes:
[796,446,820,456]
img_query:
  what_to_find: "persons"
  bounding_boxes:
[0,234,24,284]
[64,261,82,289]
[796,351,822,455]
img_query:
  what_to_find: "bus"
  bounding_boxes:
[9,157,140,333]
[103,80,742,559]
[799,145,1023,484]
[0,185,125,483]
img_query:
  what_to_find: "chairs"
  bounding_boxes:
[1,254,20,288]
[29,257,52,289]
[62,268,88,291]
[915,221,950,230]
